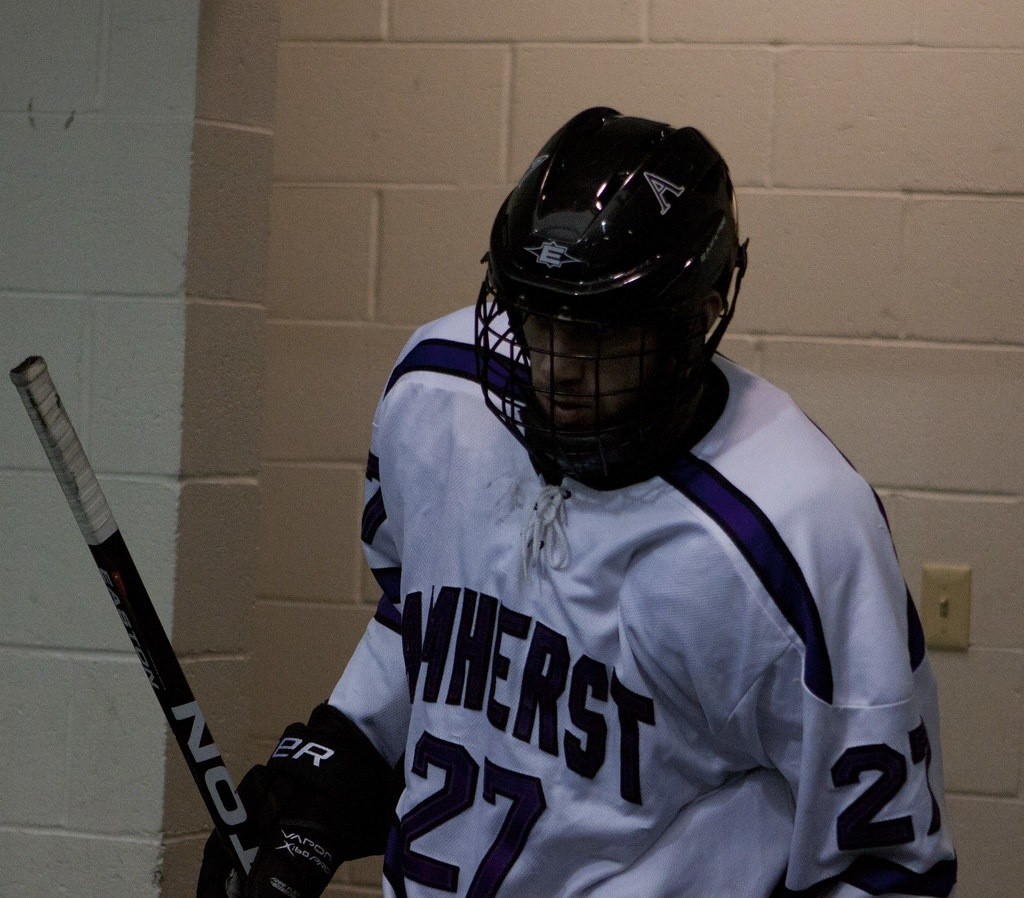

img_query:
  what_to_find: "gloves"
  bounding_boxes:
[191,706,398,897]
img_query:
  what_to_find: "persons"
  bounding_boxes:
[195,106,960,898]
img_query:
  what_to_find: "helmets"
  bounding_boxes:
[468,108,752,489]
